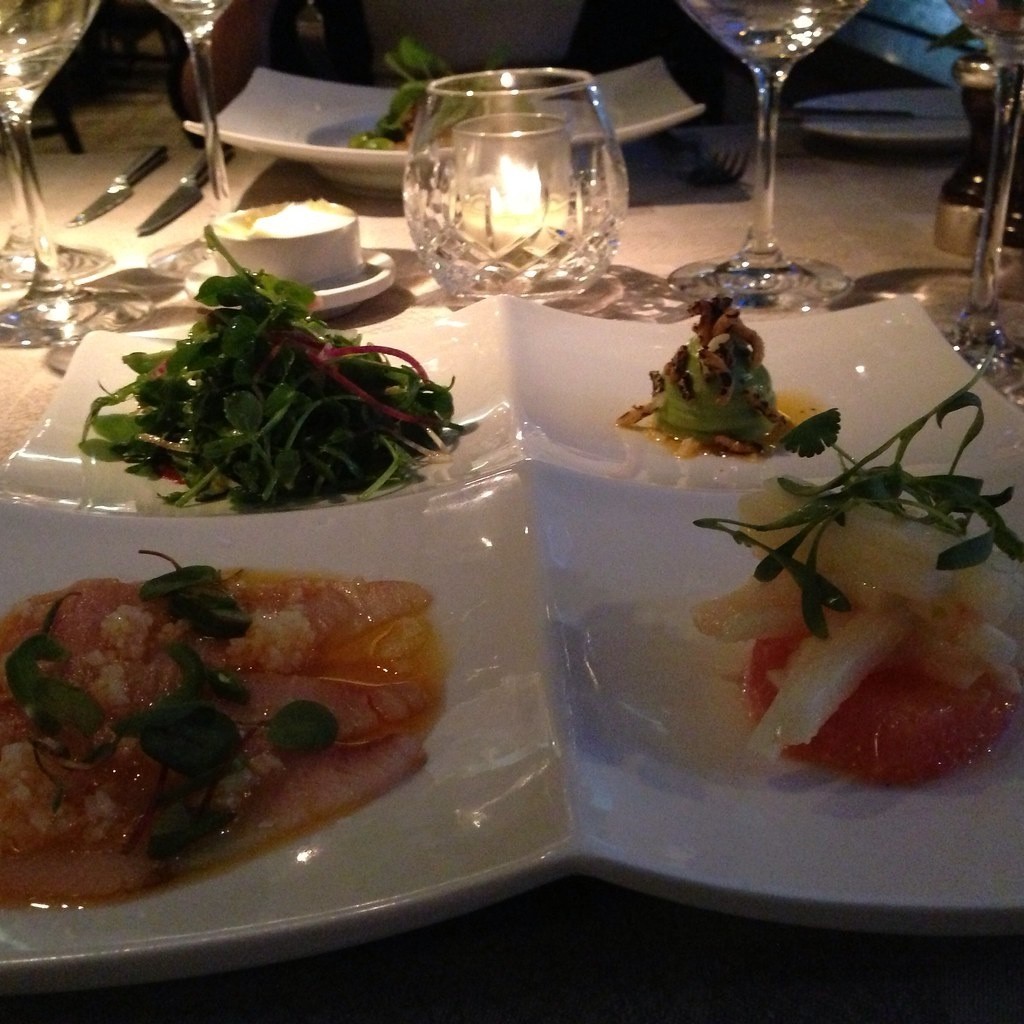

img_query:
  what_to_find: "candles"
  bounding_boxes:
[490,154,545,236]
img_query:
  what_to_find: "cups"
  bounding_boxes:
[200,197,365,293]
[403,69,626,306]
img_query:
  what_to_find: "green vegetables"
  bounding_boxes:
[79,225,463,511]
[349,38,534,152]
[692,343,1024,644]
[4,548,338,859]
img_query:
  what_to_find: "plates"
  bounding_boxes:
[187,54,705,201]
[802,88,972,143]
[181,241,398,317]
[1,295,1024,997]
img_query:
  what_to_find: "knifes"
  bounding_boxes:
[137,143,243,236]
[67,143,172,229]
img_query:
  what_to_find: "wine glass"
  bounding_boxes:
[1,0,151,346]
[670,0,883,314]
[147,1,235,211]
[1,88,114,289]
[938,1,1023,411]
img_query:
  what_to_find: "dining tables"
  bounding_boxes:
[0,119,1024,1024]
